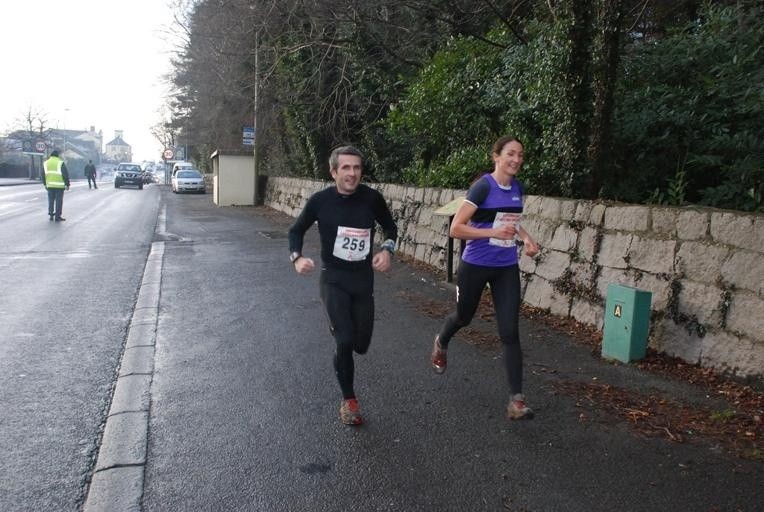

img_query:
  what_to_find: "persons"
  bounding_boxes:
[84,159,98,189]
[286,146,398,426]
[41,151,70,221]
[431,136,539,420]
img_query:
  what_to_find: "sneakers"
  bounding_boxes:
[507,393,535,421]
[431,333,449,375]
[339,399,363,425]
[49,217,66,221]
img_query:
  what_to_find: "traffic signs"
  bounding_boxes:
[34,140,47,152]
[164,149,174,159]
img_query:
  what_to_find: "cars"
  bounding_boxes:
[170,160,206,193]
[100,168,111,179]
[114,162,160,190]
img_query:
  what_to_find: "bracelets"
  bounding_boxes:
[380,242,394,257]
[289,251,301,263]
